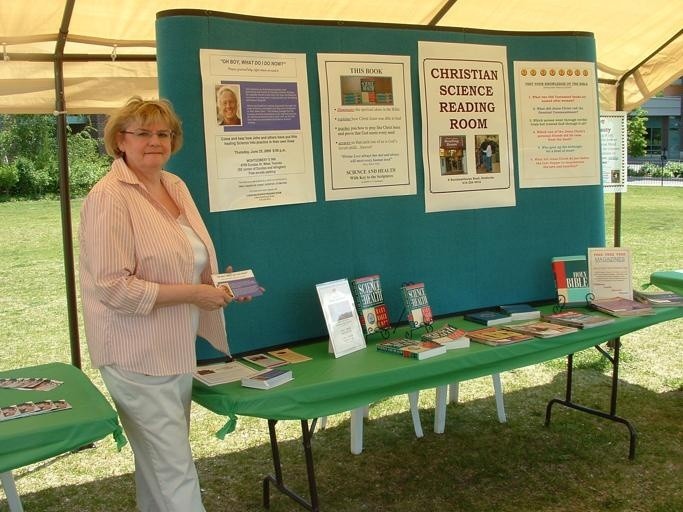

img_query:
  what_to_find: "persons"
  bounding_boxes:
[218,284,233,298]
[76,94,265,511]
[479,137,496,173]
[0,377,68,419]
[216,88,240,125]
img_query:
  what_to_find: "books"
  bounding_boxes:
[240,367,293,390]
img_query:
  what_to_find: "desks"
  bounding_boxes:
[193,271,682,511]
[1,363,128,511]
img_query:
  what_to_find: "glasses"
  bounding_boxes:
[120,129,173,141]
